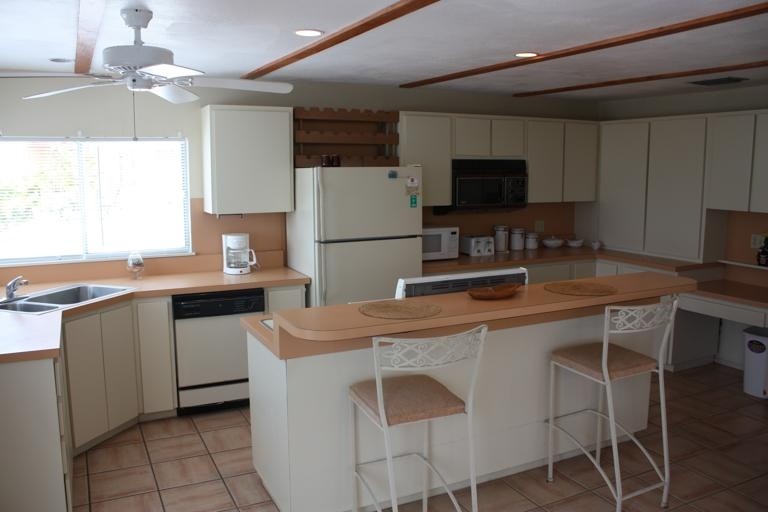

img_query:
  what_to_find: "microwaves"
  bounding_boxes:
[422,226,460,260]
[450,159,526,207]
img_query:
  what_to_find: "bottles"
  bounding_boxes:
[494,224,539,250]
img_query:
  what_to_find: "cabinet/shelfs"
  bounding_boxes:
[199,103,298,216]
[398,109,454,205]
[750,110,766,215]
[597,119,649,252]
[452,114,492,157]
[563,122,600,201]
[527,122,565,203]
[704,112,756,211]
[0,279,307,512]
[491,119,526,155]
[644,116,707,259]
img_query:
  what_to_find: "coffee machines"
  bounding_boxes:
[221,232,255,274]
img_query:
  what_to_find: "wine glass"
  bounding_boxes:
[125,253,145,281]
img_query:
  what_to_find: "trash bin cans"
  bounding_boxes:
[741,326,768,399]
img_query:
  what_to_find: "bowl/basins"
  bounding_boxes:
[564,239,584,247]
[467,283,522,301]
[542,238,564,248]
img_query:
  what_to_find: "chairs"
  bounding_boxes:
[349,322,489,511]
[541,290,682,511]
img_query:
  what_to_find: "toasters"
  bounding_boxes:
[460,235,494,258]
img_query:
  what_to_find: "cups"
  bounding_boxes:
[319,155,330,167]
[331,154,341,167]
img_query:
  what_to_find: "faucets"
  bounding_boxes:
[4,275,30,301]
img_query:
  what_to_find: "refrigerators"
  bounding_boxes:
[285,165,426,309]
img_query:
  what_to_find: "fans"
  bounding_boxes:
[18,5,291,108]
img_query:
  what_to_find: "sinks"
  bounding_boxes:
[0,301,64,314]
[24,283,136,314]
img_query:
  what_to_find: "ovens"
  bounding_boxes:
[171,289,267,412]
